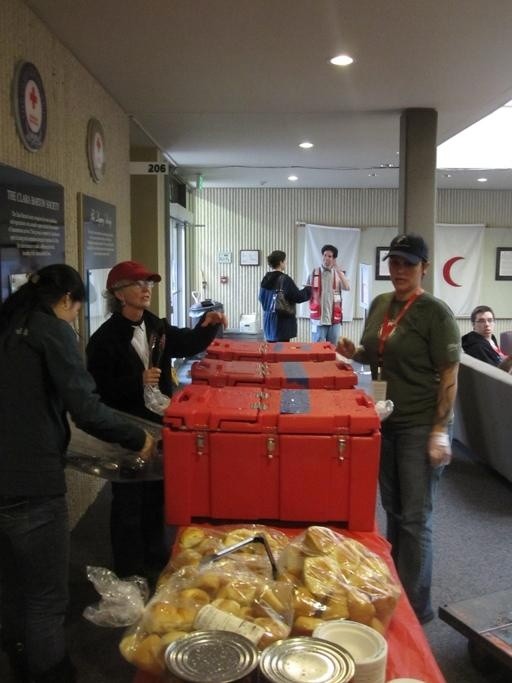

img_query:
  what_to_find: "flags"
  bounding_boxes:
[300,226,360,323]
[433,226,484,317]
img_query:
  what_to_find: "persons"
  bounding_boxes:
[334,234,463,625]
[258,250,313,343]
[86,261,227,578]
[305,245,351,345]
[2,262,157,683]
[463,306,512,374]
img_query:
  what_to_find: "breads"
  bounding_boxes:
[370,618,386,634]
[295,616,323,635]
[117,525,292,632]
[160,631,187,646]
[277,526,397,618]
[133,635,164,676]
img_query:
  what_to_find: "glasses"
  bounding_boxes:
[117,279,154,292]
[474,318,494,324]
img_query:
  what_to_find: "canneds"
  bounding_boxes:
[165,630,259,683]
[311,620,388,682]
[259,636,356,683]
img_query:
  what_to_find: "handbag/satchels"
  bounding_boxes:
[269,274,296,317]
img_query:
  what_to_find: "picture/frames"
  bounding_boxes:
[239,250,259,266]
[495,247,512,281]
[374,246,392,280]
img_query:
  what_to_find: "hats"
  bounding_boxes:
[106,260,161,289]
[382,234,428,266]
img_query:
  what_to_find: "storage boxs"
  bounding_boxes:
[162,385,382,532]
[191,360,358,388]
[205,339,336,361]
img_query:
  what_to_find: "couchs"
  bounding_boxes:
[450,353,511,491]
